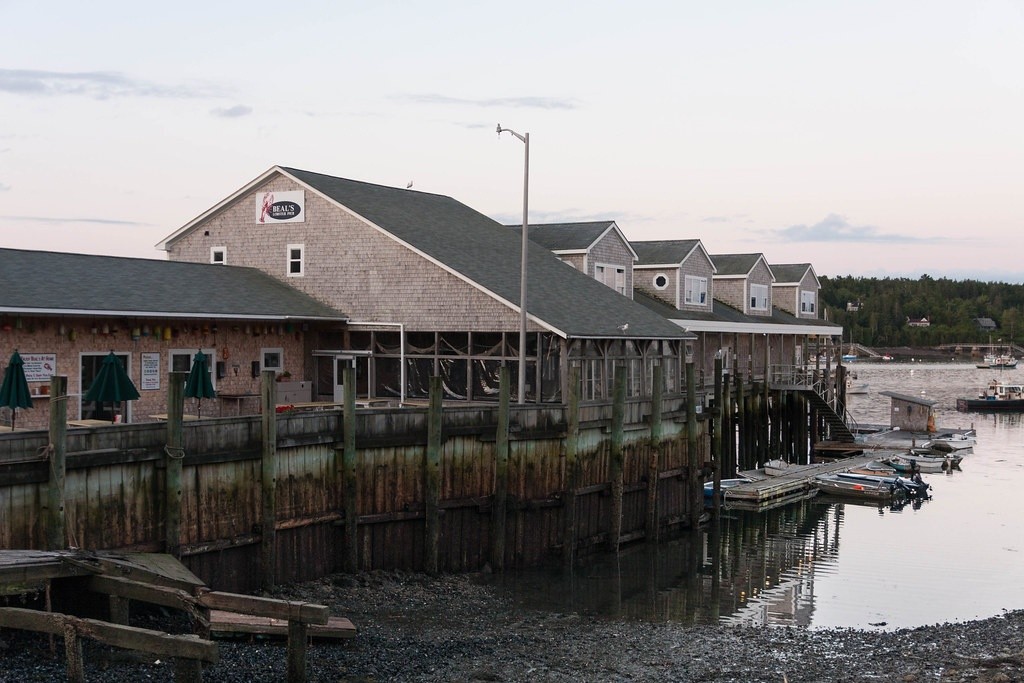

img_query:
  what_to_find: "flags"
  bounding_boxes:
[998,339,1002,342]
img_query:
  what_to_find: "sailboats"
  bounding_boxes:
[809,308,837,363]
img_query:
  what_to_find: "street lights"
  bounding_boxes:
[496,123,530,402]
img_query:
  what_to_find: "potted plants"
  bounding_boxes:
[276,371,292,382]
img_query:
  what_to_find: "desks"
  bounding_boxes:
[0,425,31,433]
[218,394,261,417]
[66,419,125,426]
[9,393,85,398]
[148,414,210,421]
[275,401,344,410]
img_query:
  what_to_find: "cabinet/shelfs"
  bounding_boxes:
[275,381,312,403]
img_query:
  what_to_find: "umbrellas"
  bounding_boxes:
[84,350,140,422]
[183,348,216,418]
[0,350,34,430]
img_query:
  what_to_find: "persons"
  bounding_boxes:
[910,459,915,481]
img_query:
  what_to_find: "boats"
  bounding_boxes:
[956,378,1024,412]
[984,353,1018,368]
[865,461,896,473]
[931,436,978,450]
[889,453,952,470]
[845,361,869,395]
[815,476,917,502]
[909,448,963,472]
[870,354,894,362]
[976,364,990,369]
[835,472,929,495]
[849,468,898,476]
[842,354,857,362]
